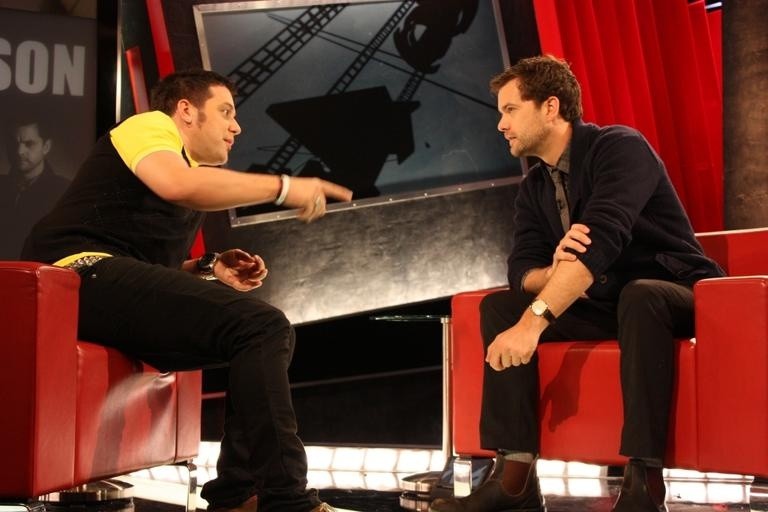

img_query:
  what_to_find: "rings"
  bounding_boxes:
[314,197,323,205]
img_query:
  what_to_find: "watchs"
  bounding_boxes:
[195,250,220,279]
[529,297,558,326]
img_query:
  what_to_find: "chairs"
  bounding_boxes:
[0,256,207,511]
[446,227,768,510]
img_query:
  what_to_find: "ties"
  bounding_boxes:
[551,168,570,237]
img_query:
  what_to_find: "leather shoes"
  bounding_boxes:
[604,458,668,511]
[424,450,549,511]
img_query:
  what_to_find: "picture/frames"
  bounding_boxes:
[193,2,533,227]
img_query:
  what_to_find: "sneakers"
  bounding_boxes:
[206,492,361,511]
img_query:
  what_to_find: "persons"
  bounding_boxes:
[425,52,731,511]
[18,68,356,512]
[1,108,74,265]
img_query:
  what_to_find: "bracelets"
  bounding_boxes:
[276,172,290,206]
